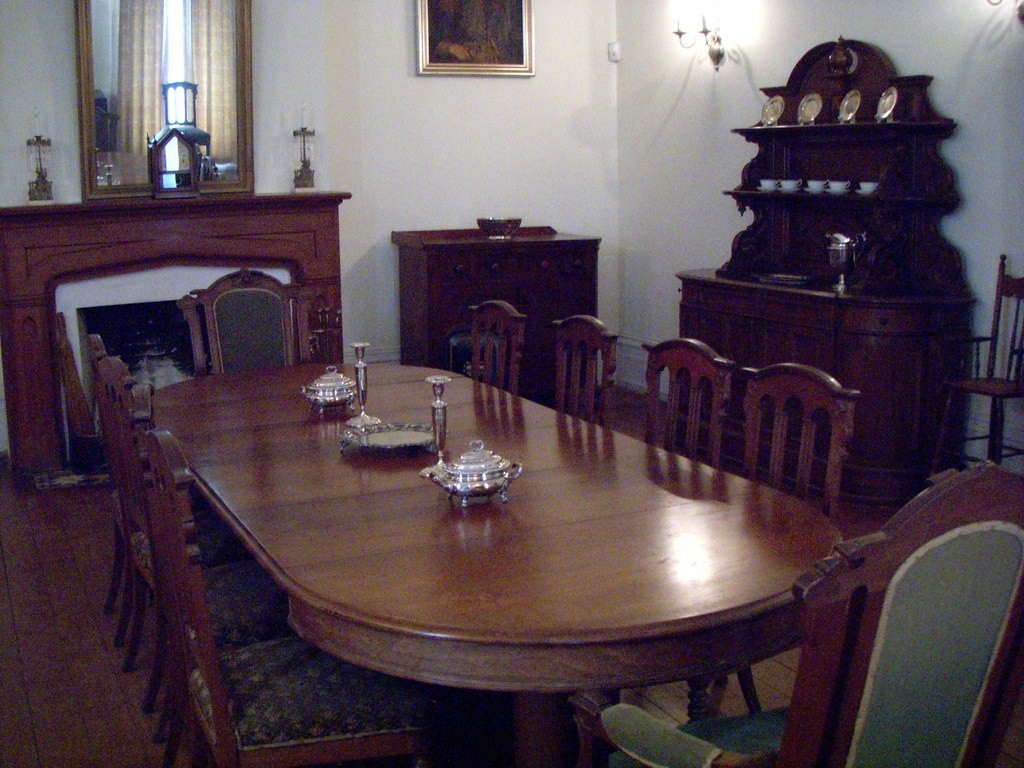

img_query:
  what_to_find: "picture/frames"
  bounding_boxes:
[413,0,535,77]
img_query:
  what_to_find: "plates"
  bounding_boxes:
[856,190,874,195]
[804,188,825,195]
[798,92,822,122]
[757,186,778,192]
[753,274,810,285]
[840,89,861,122]
[762,95,785,124]
[339,422,437,456]
[826,189,850,195]
[778,187,801,192]
[877,87,898,119]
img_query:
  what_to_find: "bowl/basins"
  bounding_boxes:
[477,217,522,240]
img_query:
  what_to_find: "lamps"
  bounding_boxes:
[671,0,725,72]
[987,1,1024,22]
[24,113,54,201]
[293,107,316,190]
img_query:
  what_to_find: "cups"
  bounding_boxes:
[759,179,781,188]
[780,179,802,188]
[860,182,879,190]
[807,180,830,189]
[828,181,850,190]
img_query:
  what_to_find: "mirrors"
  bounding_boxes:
[74,0,254,201]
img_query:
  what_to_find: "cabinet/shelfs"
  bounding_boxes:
[1,190,352,492]
[674,35,977,508]
[390,226,602,405]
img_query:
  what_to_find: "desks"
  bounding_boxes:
[150,362,843,767]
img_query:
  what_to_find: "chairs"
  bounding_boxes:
[90,254,1024,768]
[91,82,240,189]
